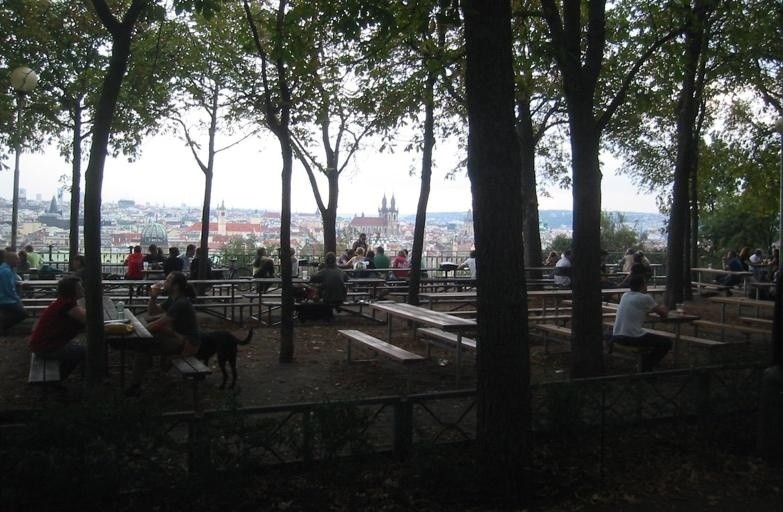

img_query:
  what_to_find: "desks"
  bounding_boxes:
[77,297,152,393]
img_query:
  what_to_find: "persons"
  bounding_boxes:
[714,240,780,297]
[0,241,44,337]
[609,276,669,372]
[456,249,477,280]
[545,250,560,278]
[121,242,215,295]
[310,250,348,314]
[62,255,85,285]
[337,232,428,293]
[289,246,298,277]
[30,276,87,380]
[600,248,609,280]
[610,247,653,286]
[124,271,200,396]
[554,250,572,286]
[250,246,275,294]
[312,262,325,303]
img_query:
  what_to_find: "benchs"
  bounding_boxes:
[330,261,782,392]
[162,355,212,410]
[28,352,64,409]
[4,260,321,330]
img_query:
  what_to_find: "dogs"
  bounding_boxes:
[196,326,254,390]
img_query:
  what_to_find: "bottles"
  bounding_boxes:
[115,300,125,320]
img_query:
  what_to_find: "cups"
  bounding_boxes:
[150,280,165,294]
[143,261,148,271]
[708,264,713,270]
[763,258,767,264]
[675,303,686,316]
[22,274,30,281]
[302,271,308,280]
